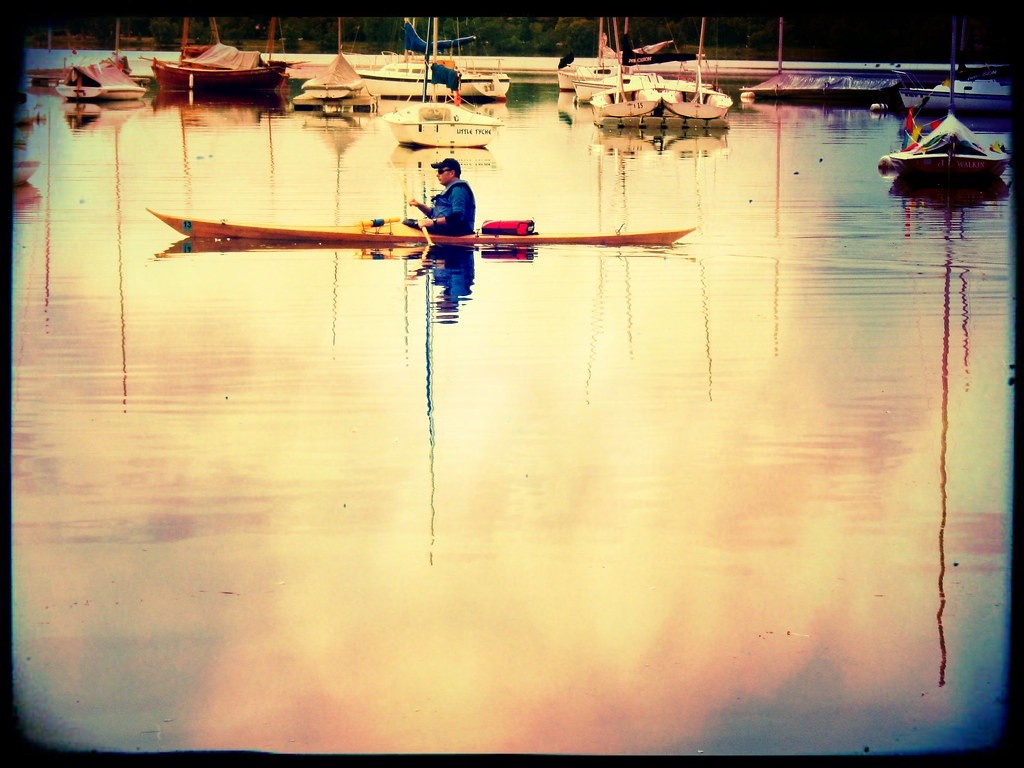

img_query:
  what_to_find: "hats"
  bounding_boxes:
[431,158,461,174]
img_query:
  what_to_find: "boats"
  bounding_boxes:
[301,17,367,99]
[145,206,699,244]
[52,18,146,100]
[880,114,1009,184]
[15,127,41,184]
[900,69,1010,113]
[150,18,289,91]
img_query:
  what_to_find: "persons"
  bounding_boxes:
[409,158,476,237]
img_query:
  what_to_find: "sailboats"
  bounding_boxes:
[573,16,713,106]
[662,17,733,118]
[594,17,662,118]
[558,15,657,93]
[357,17,511,99]
[383,18,503,149]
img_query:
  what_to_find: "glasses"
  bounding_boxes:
[438,169,452,174]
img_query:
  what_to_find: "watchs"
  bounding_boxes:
[432,218,437,226]
